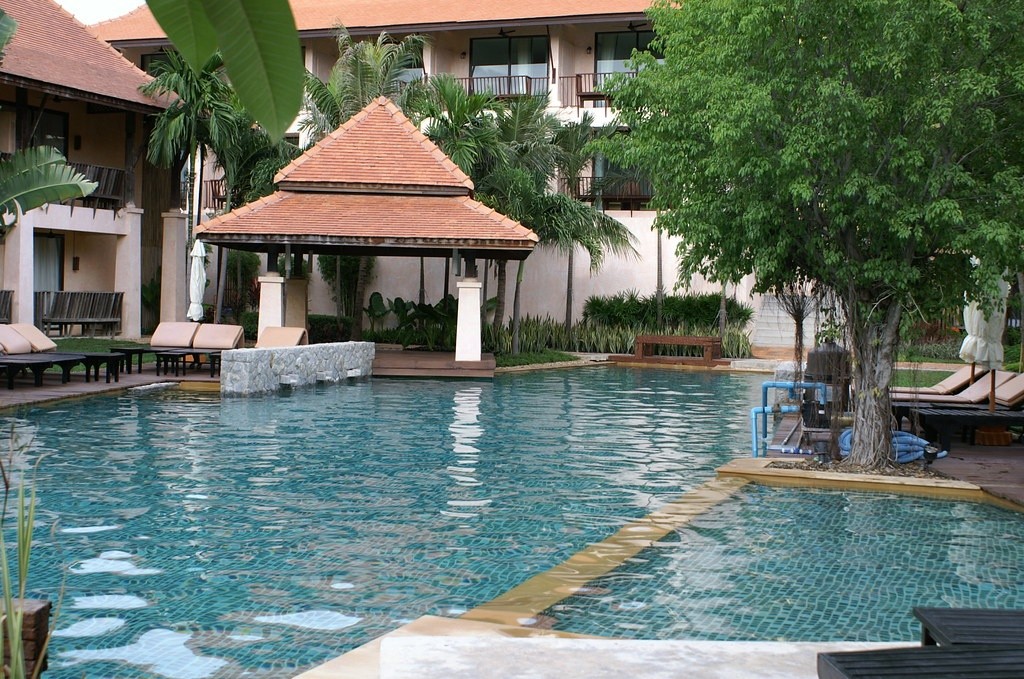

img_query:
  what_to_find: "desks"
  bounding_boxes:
[85,352,120,383]
[813,604,1024,679]
[110,348,143,375]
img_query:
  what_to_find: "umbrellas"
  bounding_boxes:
[959,254,1011,413]
[186,238,207,362]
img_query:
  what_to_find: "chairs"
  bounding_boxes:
[110,322,202,373]
[156,324,244,377]
[10,323,123,384]
[848,366,1024,436]
[0,324,87,386]
[209,327,309,377]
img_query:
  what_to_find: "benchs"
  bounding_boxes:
[0,289,125,339]
[557,174,655,209]
[0,151,124,221]
[635,333,723,359]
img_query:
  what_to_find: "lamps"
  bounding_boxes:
[460,52,466,58]
[587,45,592,54]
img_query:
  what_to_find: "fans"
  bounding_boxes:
[484,27,516,40]
[608,21,647,32]
